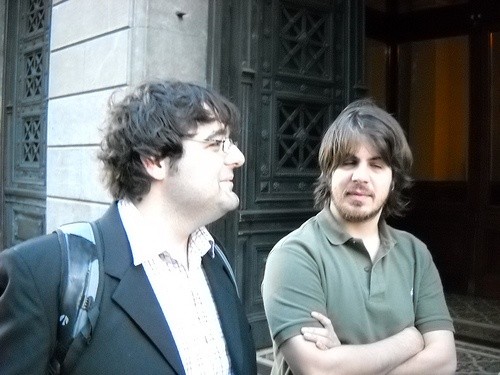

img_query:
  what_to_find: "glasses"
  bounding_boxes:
[179,137,239,152]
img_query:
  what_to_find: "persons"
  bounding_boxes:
[261,96,457,375]
[0,78,256,375]
